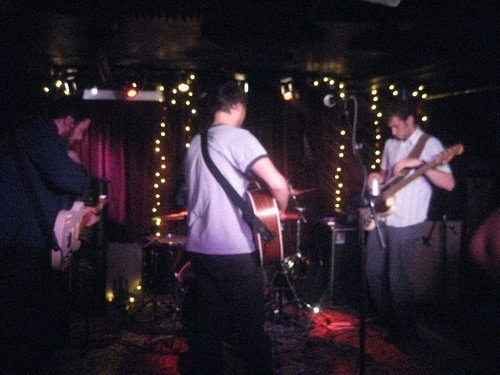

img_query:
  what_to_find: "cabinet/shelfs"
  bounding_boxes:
[67,200,109,316]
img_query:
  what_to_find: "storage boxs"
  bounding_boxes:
[312,215,360,308]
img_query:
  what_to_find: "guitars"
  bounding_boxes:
[52,194,108,270]
[352,143,465,229]
[243,188,284,270]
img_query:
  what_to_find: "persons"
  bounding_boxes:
[184,81,289,375]
[0,94,109,375]
[364,99,454,344]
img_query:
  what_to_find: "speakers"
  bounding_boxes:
[107,240,144,296]
[410,218,463,313]
[309,223,364,308]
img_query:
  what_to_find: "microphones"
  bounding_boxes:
[422,236,431,247]
[323,94,351,108]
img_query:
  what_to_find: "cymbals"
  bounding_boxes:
[144,209,189,222]
[281,212,300,220]
[289,188,319,195]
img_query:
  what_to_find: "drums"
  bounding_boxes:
[146,232,189,276]
